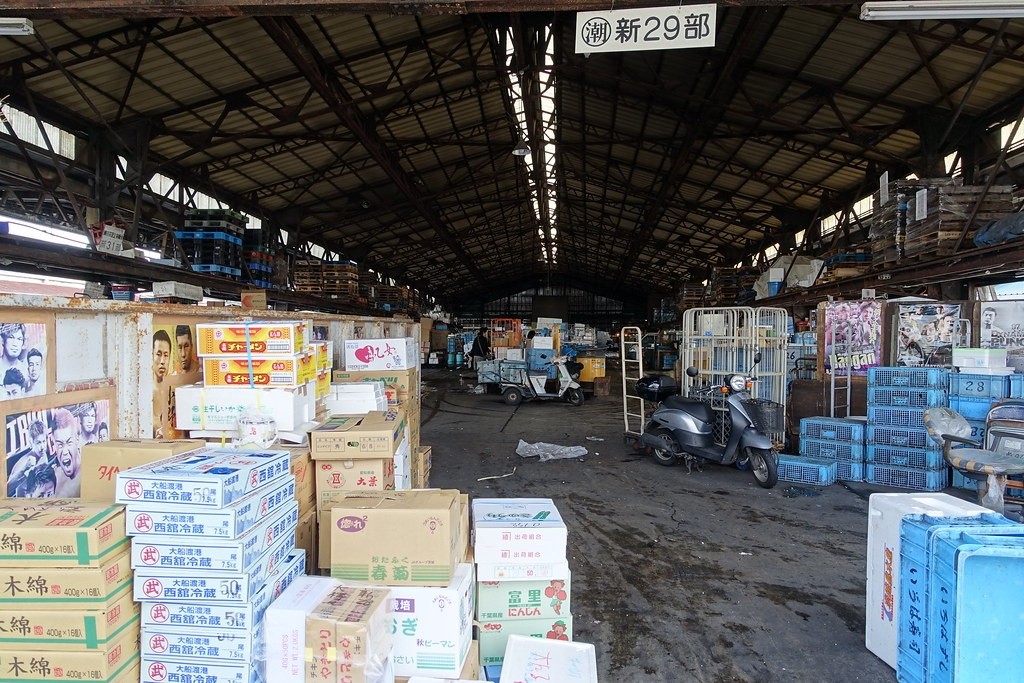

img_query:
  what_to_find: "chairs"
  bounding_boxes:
[923,407,1024,515]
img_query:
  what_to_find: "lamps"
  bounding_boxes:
[512,70,530,155]
[362,199,371,208]
[673,235,689,245]
[537,251,544,261]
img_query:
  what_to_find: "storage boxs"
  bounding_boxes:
[153,281,203,306]
[207,301,225,306]
[111,284,133,300]
[646,314,1024,683]
[766,268,787,296]
[0,316,611,683]
[241,289,267,309]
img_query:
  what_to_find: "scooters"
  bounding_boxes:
[635,352,785,489]
[497,343,584,407]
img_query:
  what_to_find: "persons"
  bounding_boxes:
[470,327,491,364]
[176,325,199,374]
[899,305,961,365]
[153,329,172,382]
[825,301,881,347]
[0,323,42,400]
[981,307,1002,332]
[7,402,108,498]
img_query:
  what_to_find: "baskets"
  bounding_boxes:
[740,398,785,433]
